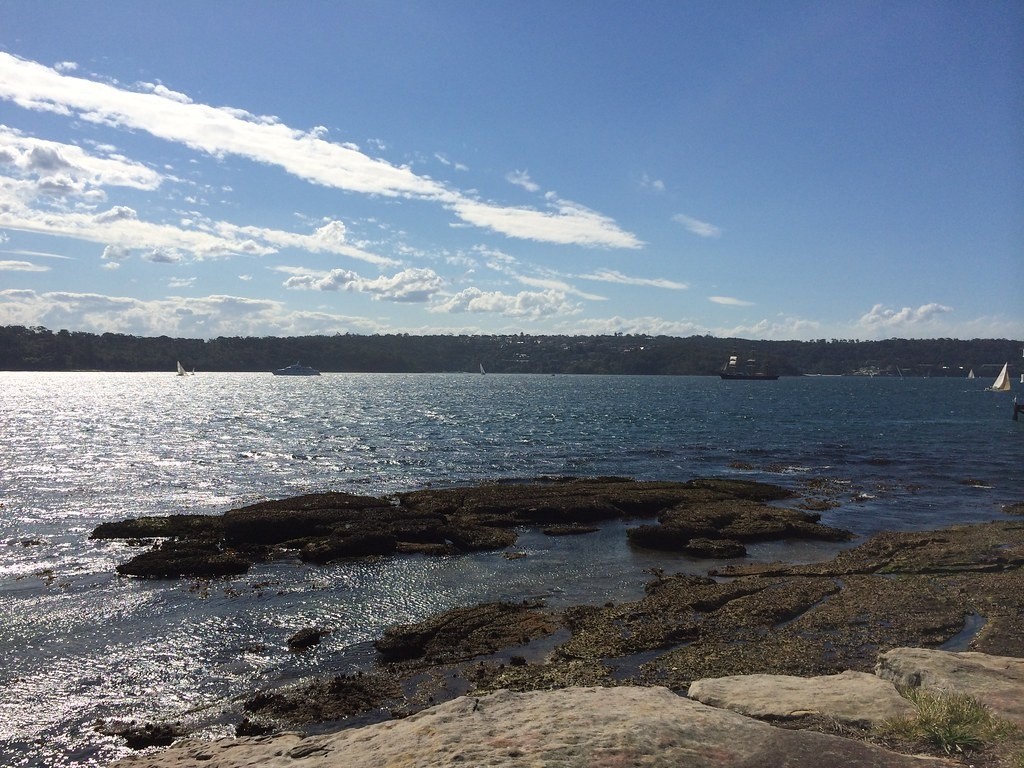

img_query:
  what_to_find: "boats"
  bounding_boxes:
[272,361,321,376]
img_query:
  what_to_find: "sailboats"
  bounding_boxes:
[720,356,779,380]
[984,361,1011,392]
[176,361,188,376]
[480,363,485,375]
[190,368,195,375]
[966,369,975,379]
